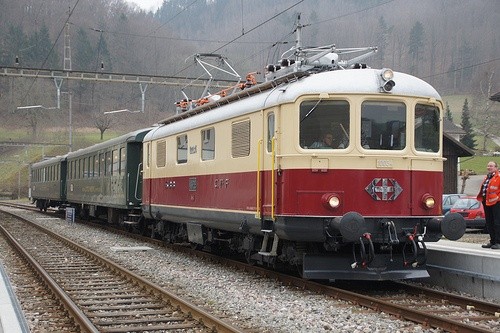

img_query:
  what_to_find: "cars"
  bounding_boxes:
[441,193,487,230]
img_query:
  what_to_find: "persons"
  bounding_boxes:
[310,127,370,149]
[476,160,500,249]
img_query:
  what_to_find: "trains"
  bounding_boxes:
[28,47,467,290]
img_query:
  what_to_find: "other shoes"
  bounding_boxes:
[491,243,500,249]
[482,243,494,248]
